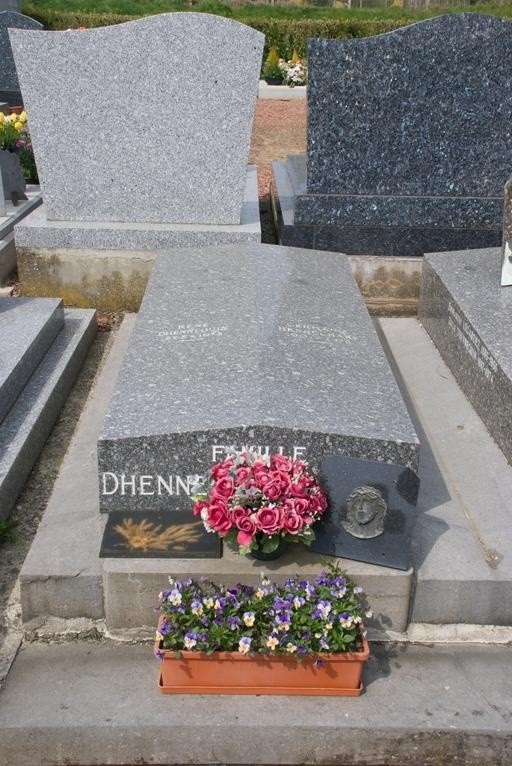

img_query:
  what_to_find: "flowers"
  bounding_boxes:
[157,560,375,666]
[194,453,327,552]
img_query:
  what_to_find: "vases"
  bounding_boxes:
[248,533,286,559]
[153,615,370,696]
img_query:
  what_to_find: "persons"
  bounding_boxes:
[340,485,387,540]
